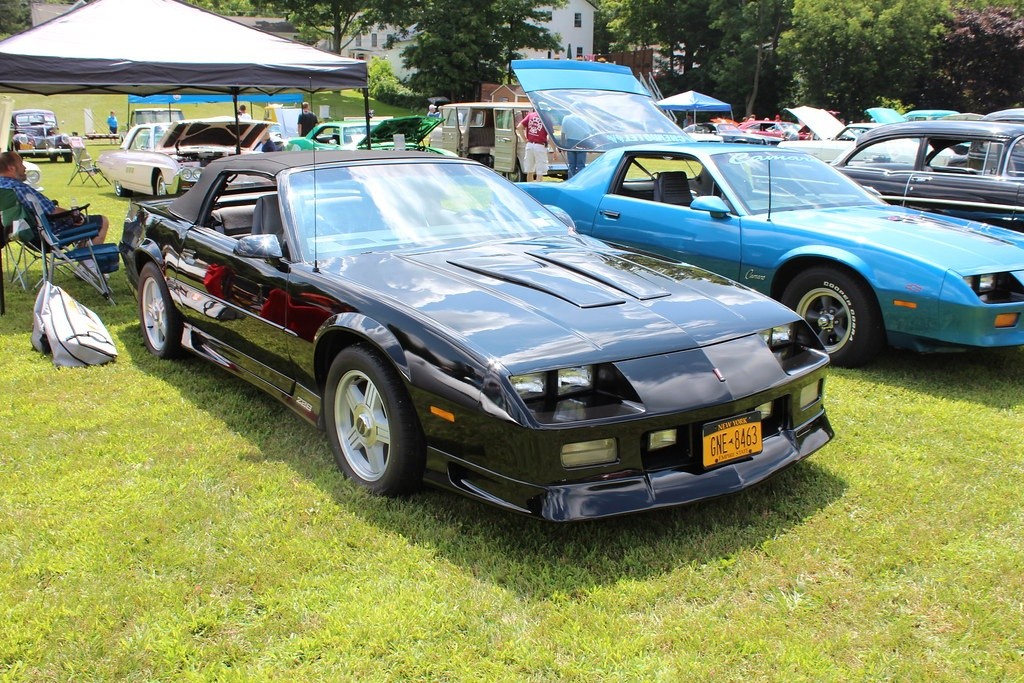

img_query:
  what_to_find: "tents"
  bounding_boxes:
[0,0,371,315]
[655,91,734,133]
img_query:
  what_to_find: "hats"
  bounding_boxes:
[429,104,437,109]
[539,102,551,112]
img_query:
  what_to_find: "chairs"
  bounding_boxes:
[251,194,287,259]
[67,136,111,187]
[333,135,347,146]
[712,182,721,197]
[0,188,90,295]
[652,171,693,209]
[32,195,118,309]
[319,105,331,119]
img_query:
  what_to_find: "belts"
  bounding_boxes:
[530,141,543,145]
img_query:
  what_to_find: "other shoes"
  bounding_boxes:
[74,264,110,281]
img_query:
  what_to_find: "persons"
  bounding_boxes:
[298,103,318,136]
[683,113,693,129]
[0,151,110,280]
[68,131,92,172]
[517,102,558,182]
[739,114,782,125]
[840,118,875,125]
[427,104,443,118]
[252,132,277,152]
[107,111,118,144]
[561,101,593,180]
[454,110,463,126]
[238,105,252,122]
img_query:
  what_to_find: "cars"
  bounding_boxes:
[682,123,782,145]
[7,109,73,163]
[829,120,1024,235]
[494,59,1024,369]
[429,101,605,182]
[864,108,971,144]
[96,116,281,197]
[946,108,1024,178]
[119,109,186,149]
[738,111,840,141]
[283,116,459,156]
[776,106,953,167]
[119,150,834,523]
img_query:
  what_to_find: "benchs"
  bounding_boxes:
[469,127,495,153]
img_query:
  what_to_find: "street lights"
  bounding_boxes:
[508,13,519,85]
